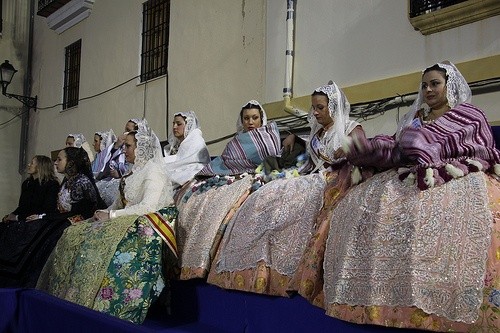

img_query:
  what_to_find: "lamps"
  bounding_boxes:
[0,60,37,112]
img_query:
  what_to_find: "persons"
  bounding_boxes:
[1,155,62,289]
[207,79,365,299]
[35,123,180,325]
[4,146,108,289]
[174,98,283,284]
[94,118,151,207]
[64,131,94,169]
[88,129,118,183]
[161,110,213,191]
[317,59,500,333]
